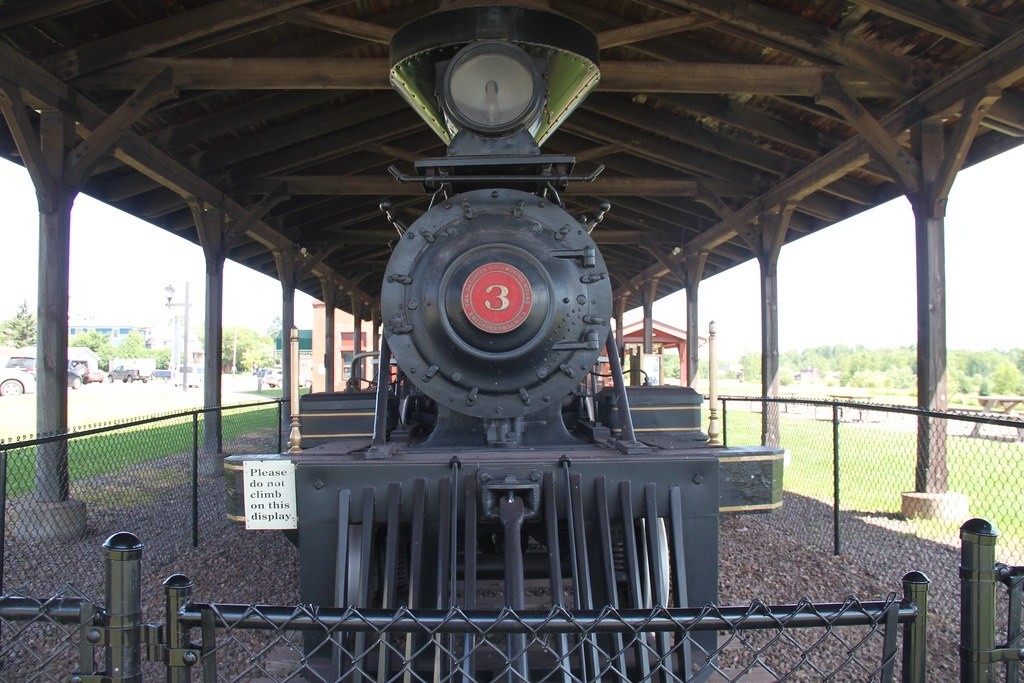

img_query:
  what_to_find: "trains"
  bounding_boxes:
[226,0,786,683]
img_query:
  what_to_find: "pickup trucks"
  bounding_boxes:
[107,364,151,383]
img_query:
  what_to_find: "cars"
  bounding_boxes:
[0,368,36,396]
[257,370,266,377]
[86,369,104,383]
[175,365,202,388]
[264,368,284,388]
[4,356,82,390]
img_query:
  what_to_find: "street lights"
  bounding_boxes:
[164,281,190,392]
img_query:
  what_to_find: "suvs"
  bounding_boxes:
[68,359,88,385]
